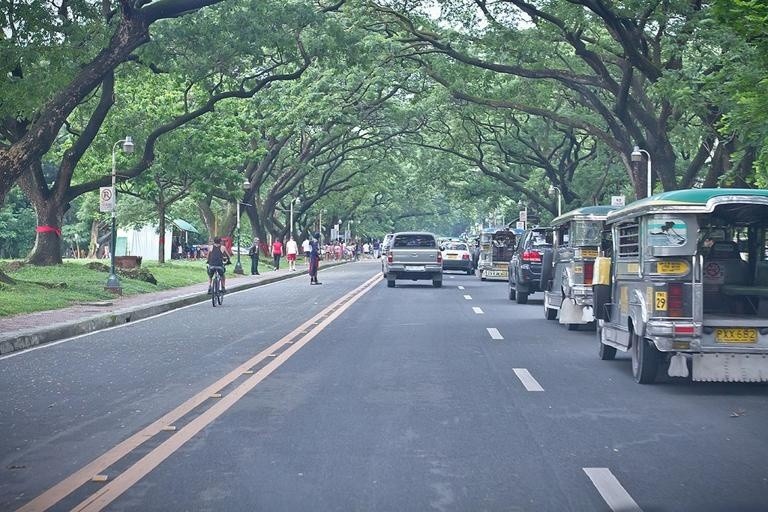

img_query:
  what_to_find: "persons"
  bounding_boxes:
[172,232,383,294]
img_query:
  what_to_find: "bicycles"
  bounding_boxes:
[207,260,227,307]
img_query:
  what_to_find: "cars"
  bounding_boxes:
[377,232,476,289]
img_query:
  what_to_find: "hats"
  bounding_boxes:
[313,231,322,239]
[213,236,223,243]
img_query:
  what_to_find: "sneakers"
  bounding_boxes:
[206,288,227,295]
[308,280,322,286]
[251,267,298,275]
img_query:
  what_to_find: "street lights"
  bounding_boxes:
[547,184,564,217]
[630,145,653,200]
[235,177,255,275]
[315,203,361,251]
[103,135,136,298]
[284,196,302,237]
